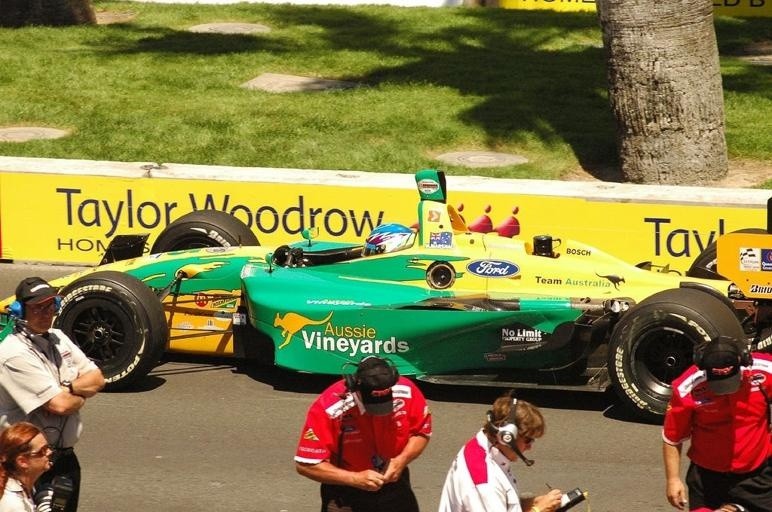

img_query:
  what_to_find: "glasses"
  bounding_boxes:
[518,433,534,444]
[19,444,47,457]
[25,302,60,315]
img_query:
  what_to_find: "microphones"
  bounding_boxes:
[13,319,30,324]
[510,444,534,466]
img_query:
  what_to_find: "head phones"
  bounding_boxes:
[346,357,399,393]
[695,338,752,371]
[9,295,62,319]
[486,395,518,447]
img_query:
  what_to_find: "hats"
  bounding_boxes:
[15,277,65,306]
[703,335,741,395]
[357,357,393,415]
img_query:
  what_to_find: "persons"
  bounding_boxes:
[1,422,56,512]
[1,277,106,512]
[436,398,562,511]
[356,222,417,261]
[295,357,432,510]
[662,335,771,511]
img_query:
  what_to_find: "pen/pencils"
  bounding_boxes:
[545,483,553,491]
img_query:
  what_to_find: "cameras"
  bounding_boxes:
[51,474,74,512]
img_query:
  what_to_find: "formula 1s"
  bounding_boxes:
[1,199,772,424]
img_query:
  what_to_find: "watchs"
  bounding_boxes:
[60,378,78,396]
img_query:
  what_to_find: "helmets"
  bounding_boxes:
[361,224,417,257]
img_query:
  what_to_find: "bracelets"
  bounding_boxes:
[529,504,541,512]
